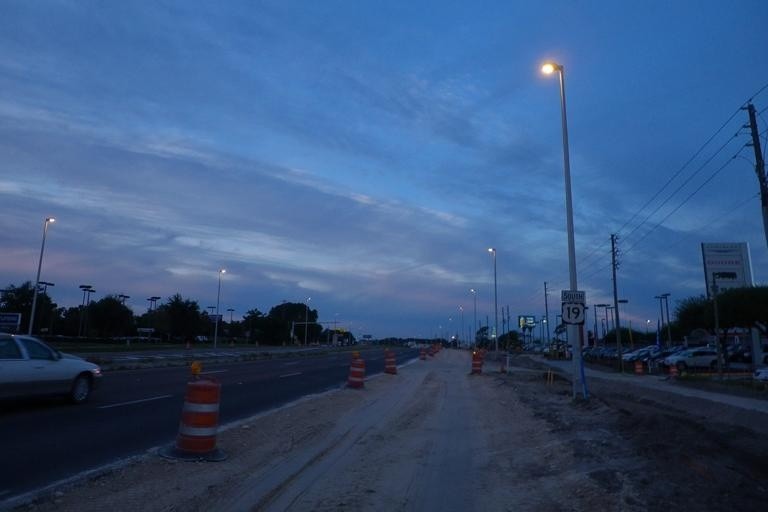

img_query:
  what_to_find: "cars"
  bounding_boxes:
[0,334,104,405]
[753,368,768,383]
[523,343,717,371]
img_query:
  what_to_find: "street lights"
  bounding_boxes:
[305,297,310,345]
[540,315,561,341]
[488,248,498,352]
[209,307,234,321]
[214,269,226,348]
[712,272,737,384]
[594,304,615,342]
[542,62,581,400]
[646,320,651,333]
[28,218,55,335]
[39,282,161,336]
[655,294,671,340]
[439,288,476,344]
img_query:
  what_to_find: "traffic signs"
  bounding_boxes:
[562,302,585,324]
